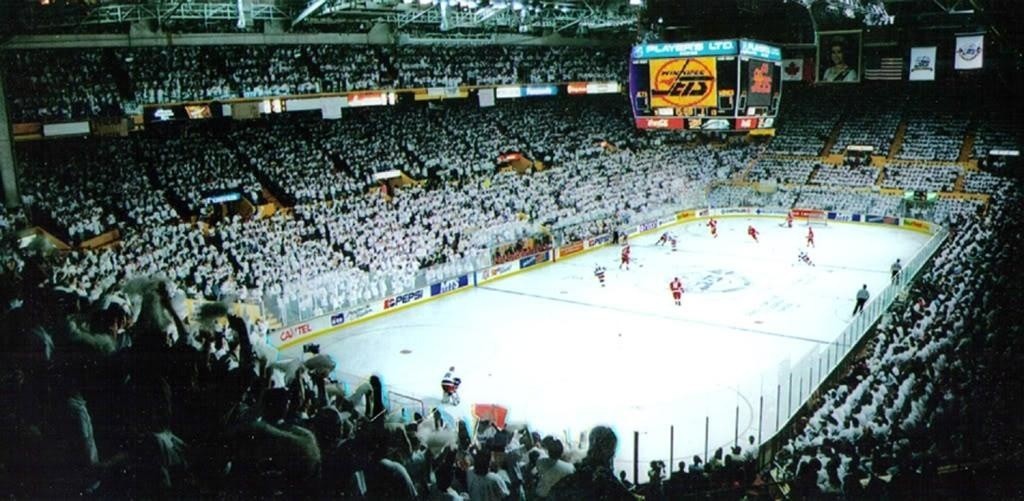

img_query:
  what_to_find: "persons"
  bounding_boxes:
[0,1,1023,500]
